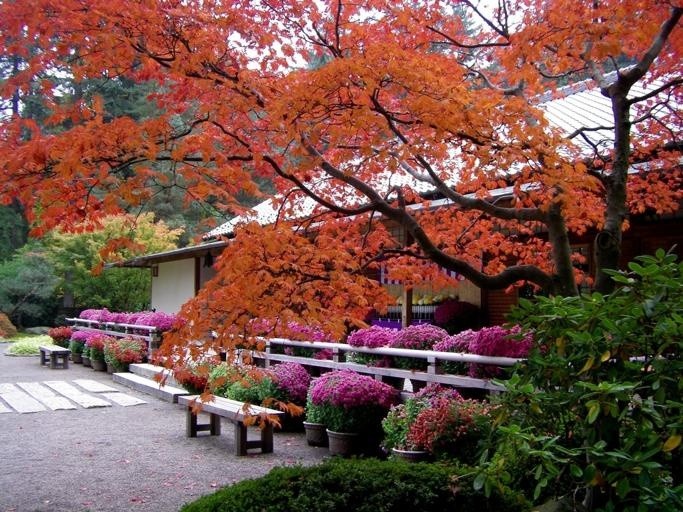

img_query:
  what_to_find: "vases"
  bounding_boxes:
[392,447,424,463]
[303,421,327,448]
[326,429,362,452]
[273,406,297,431]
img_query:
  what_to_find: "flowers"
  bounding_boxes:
[250,319,541,401]
[314,371,394,429]
[307,370,338,420]
[47,308,186,371]
[386,384,471,447]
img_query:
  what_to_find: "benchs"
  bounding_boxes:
[178,394,285,457]
[38,343,72,369]
